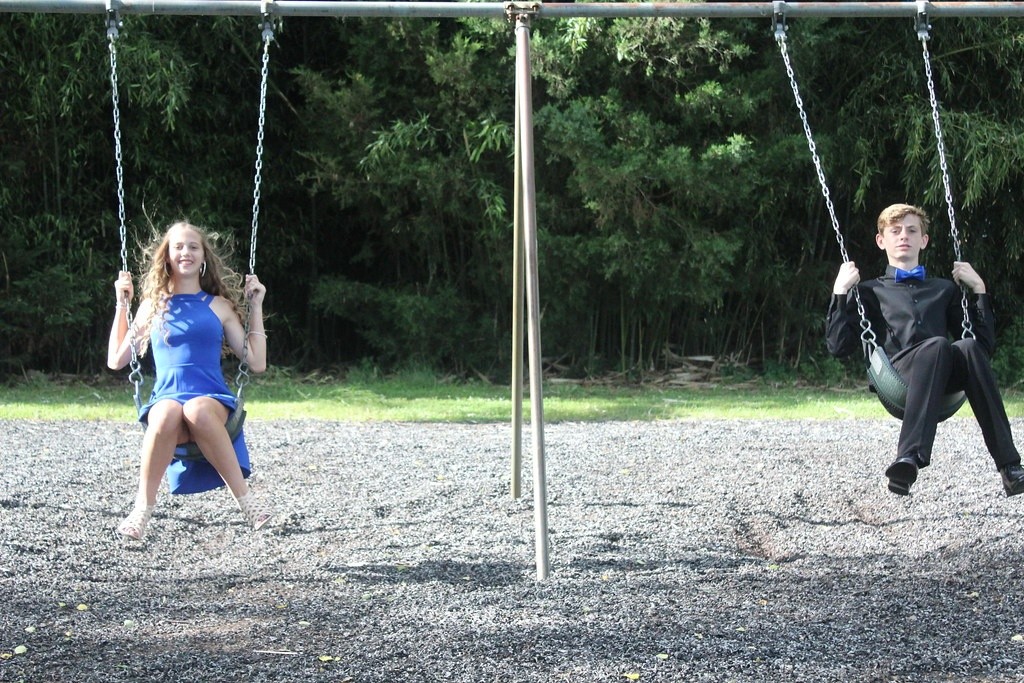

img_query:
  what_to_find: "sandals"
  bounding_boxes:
[118,504,154,541]
[235,489,273,530]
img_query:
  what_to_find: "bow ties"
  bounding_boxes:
[895,266,925,283]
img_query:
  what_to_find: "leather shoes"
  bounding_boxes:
[885,458,919,496]
[1000,462,1024,497]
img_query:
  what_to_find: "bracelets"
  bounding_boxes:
[248,330,268,339]
[114,301,132,310]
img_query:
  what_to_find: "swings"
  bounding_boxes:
[769,20,979,420]
[104,13,276,462]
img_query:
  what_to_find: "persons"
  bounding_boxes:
[825,203,1024,498]
[107,219,278,541]
